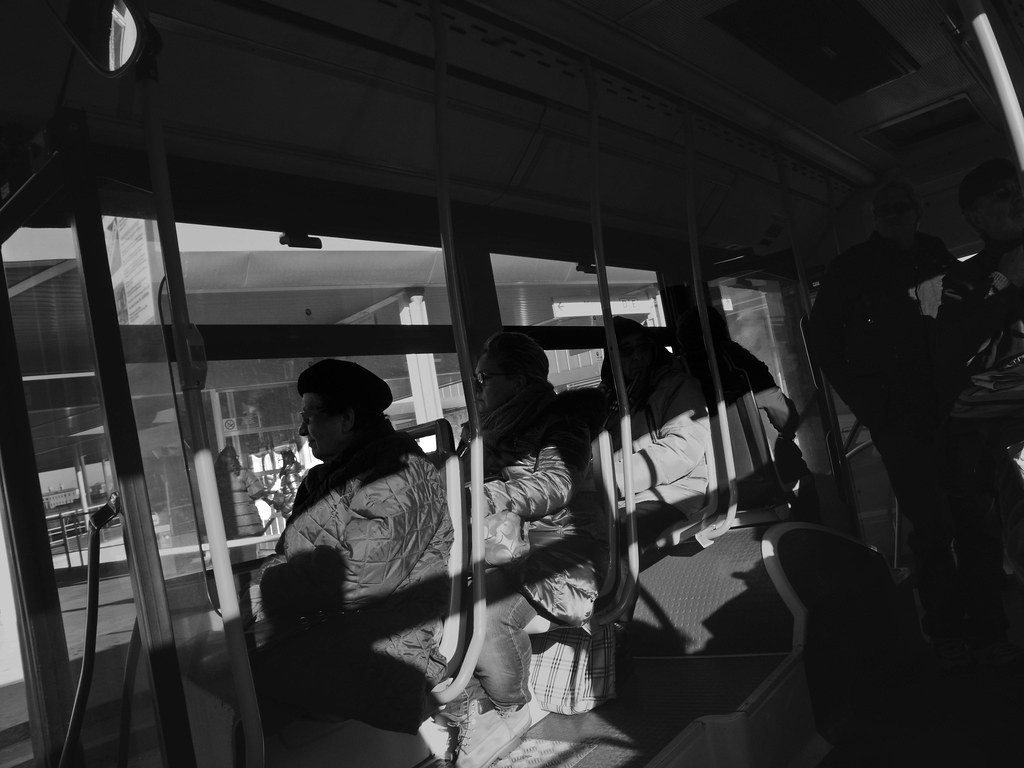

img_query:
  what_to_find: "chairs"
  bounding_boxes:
[656,404,721,548]
[399,419,486,707]
[683,359,780,494]
[560,388,624,626]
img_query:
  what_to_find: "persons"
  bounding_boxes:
[674,306,833,583]
[809,181,1024,670]
[223,358,453,768]
[217,447,264,592]
[275,451,303,519]
[933,158,1024,590]
[589,315,705,557]
[430,331,610,768]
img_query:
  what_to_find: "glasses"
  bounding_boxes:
[472,369,520,387]
[620,342,655,357]
[298,406,333,423]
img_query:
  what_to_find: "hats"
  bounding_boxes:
[297,358,394,414]
